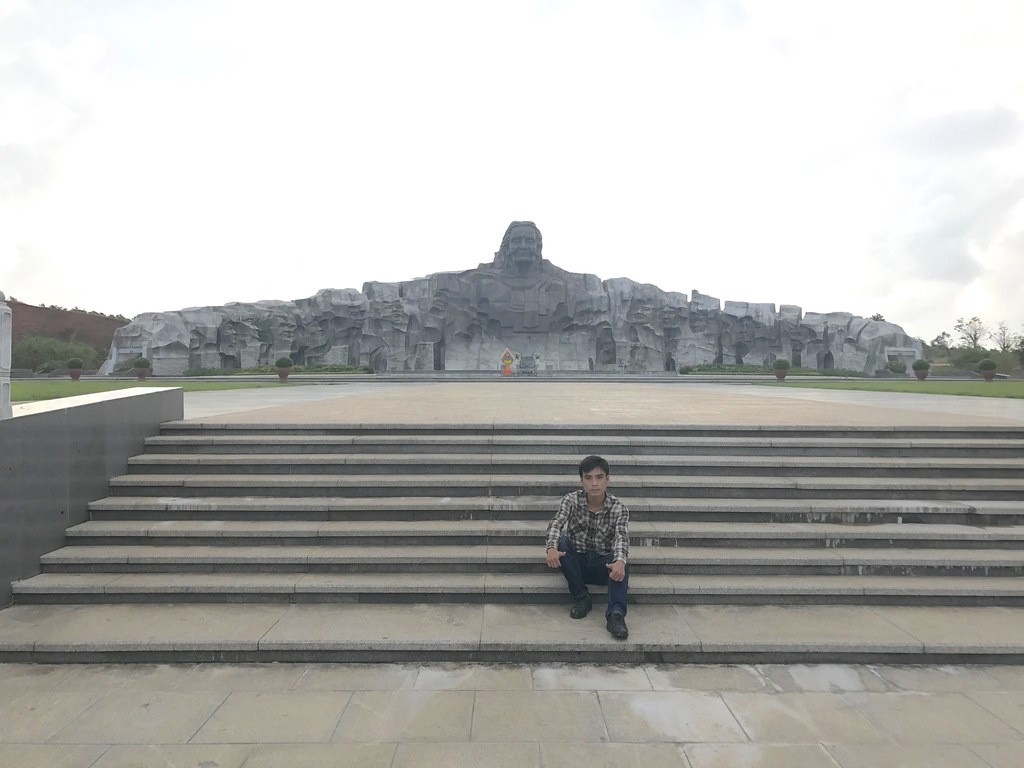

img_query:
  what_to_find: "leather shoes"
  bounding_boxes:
[570,595,592,619]
[605,611,628,638]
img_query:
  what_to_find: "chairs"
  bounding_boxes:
[544,359,561,377]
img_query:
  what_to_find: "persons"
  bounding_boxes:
[495,221,543,268]
[546,456,630,639]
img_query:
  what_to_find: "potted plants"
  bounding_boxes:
[773,359,790,383]
[133,356,150,381]
[912,359,930,382]
[276,357,292,382]
[66,357,84,381]
[978,359,997,381]
